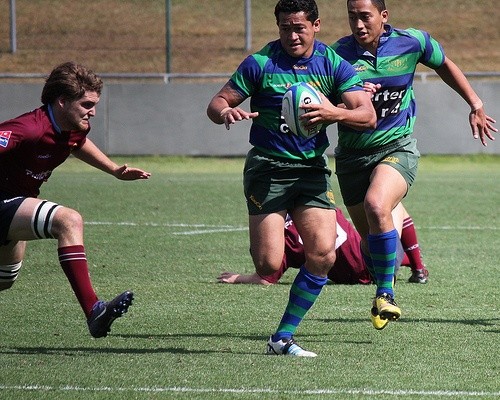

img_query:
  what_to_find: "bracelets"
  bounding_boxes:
[472,100,484,111]
[220,107,232,118]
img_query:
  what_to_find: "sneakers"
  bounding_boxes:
[371,295,402,321]
[407,270,429,285]
[86,291,135,339]
[369,307,389,330]
[268,335,317,361]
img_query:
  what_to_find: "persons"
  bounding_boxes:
[329,0,498,330]
[218,200,430,286]
[207,0,378,357]
[0,62,151,338]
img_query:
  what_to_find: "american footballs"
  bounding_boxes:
[281,82,324,141]
